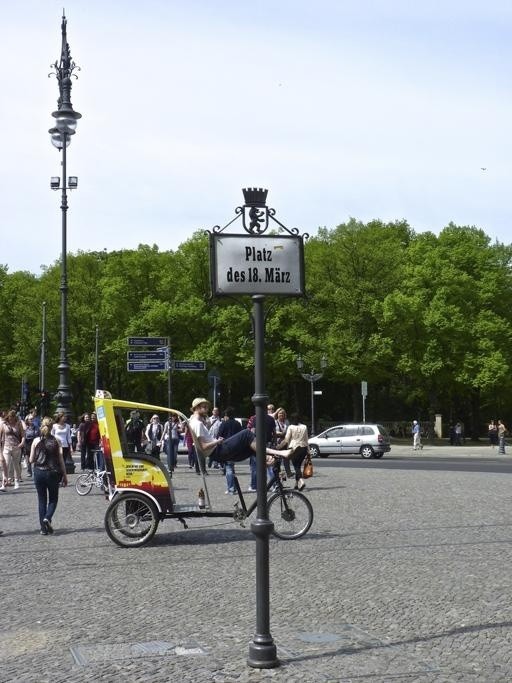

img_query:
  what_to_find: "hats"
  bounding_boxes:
[189,396,210,413]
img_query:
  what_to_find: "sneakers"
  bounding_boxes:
[224,471,305,496]
[167,464,225,476]
[0,472,33,492]
[37,517,55,536]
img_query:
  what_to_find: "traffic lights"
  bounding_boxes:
[24,400,31,415]
[40,391,47,407]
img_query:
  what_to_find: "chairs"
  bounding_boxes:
[95,407,174,513]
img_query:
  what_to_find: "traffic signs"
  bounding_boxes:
[125,360,168,371]
[171,359,207,370]
[126,336,170,346]
[155,346,168,352]
[125,349,168,362]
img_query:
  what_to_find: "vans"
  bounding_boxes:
[177,416,249,452]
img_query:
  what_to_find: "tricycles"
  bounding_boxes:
[87,397,318,550]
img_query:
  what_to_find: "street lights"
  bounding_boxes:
[40,6,84,424]
[293,351,328,437]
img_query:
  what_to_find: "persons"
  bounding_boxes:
[448,422,466,446]
[0,405,104,492]
[29,417,67,535]
[392,420,435,450]
[122,397,311,495]
[488,420,497,448]
[497,420,507,454]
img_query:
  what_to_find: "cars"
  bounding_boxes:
[305,422,392,460]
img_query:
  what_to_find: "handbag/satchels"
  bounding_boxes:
[301,453,314,479]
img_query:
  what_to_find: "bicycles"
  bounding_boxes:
[72,447,109,499]
